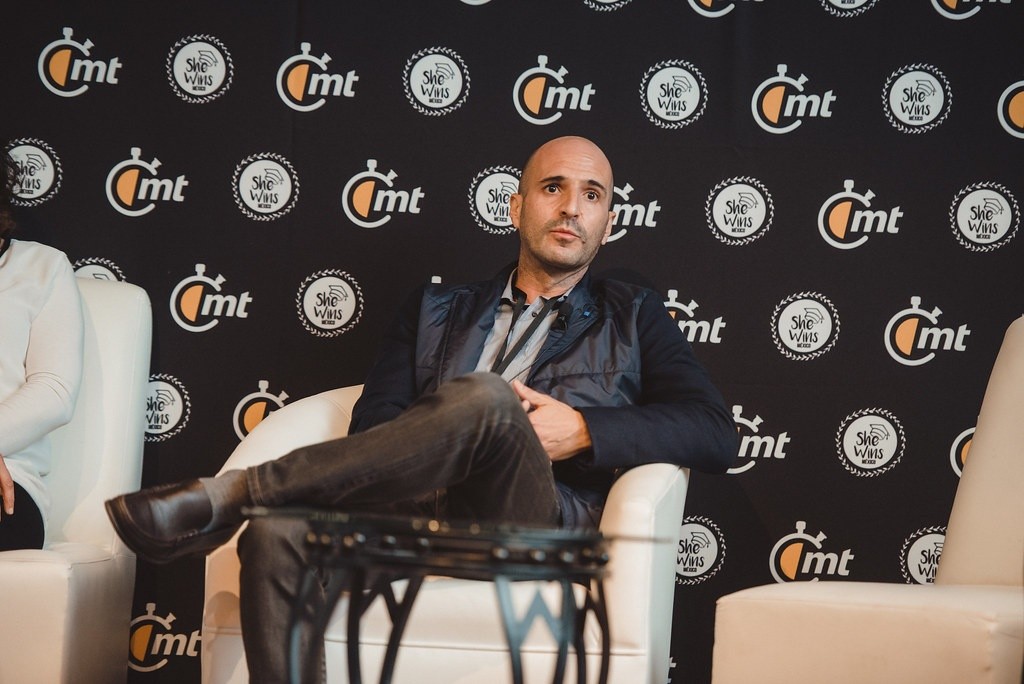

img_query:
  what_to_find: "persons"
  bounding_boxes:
[0,143,84,552]
[103,135,740,684]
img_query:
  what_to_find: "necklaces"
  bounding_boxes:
[491,287,565,376]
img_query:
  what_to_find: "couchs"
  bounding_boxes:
[200,385,691,684]
[712,316,1024,684]
[0,274,154,684]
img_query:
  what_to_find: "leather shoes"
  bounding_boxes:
[105,478,246,564]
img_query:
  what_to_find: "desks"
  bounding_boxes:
[238,505,676,684]
